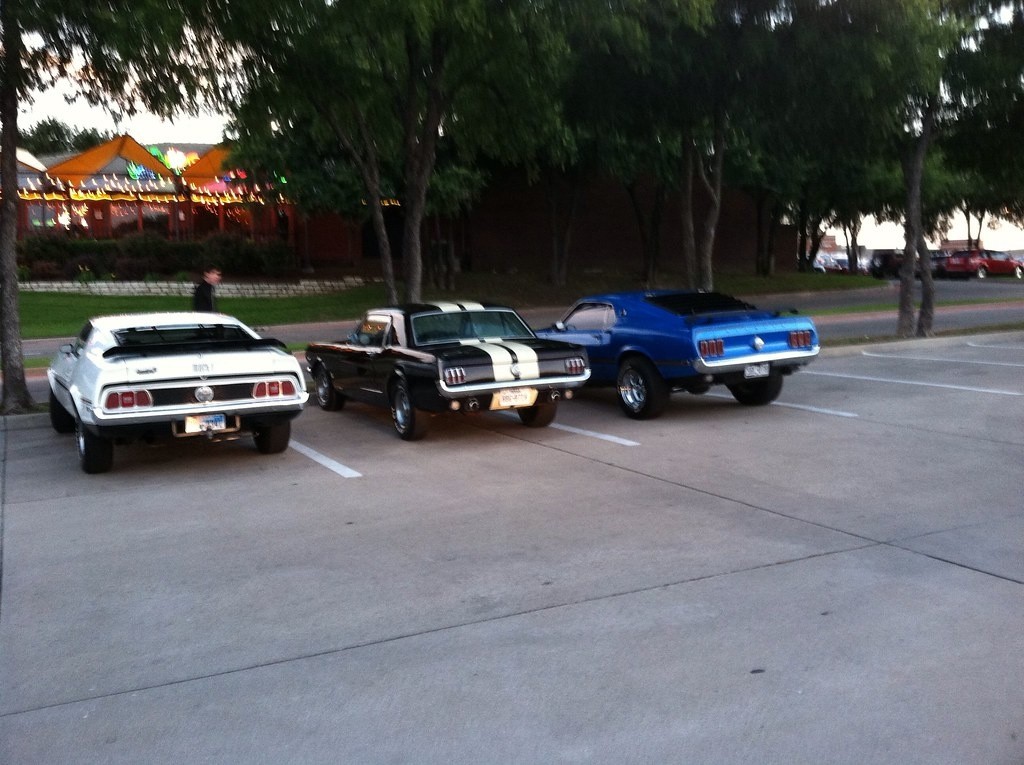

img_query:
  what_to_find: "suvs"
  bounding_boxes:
[869,247,939,280]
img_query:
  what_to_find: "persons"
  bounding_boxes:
[193,265,221,313]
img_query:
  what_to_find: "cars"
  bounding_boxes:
[307,304,590,440]
[45,311,311,473]
[807,252,869,277]
[927,250,950,278]
[534,289,820,422]
[949,249,1024,281]
